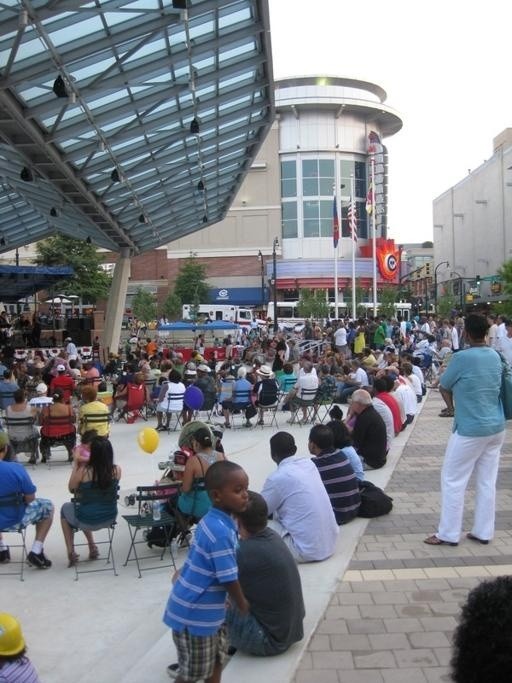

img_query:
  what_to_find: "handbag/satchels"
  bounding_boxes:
[495,349,512,420]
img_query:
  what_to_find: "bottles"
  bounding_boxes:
[153,492,160,521]
[171,538,176,565]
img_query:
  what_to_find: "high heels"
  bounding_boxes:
[423,535,459,546]
[466,532,489,544]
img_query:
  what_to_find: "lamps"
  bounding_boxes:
[86,235,93,244]
[172,0,187,9]
[190,118,200,134]
[20,166,34,182]
[50,206,62,217]
[52,74,71,98]
[138,214,148,224]
[1,237,8,246]
[203,215,207,223]
[197,180,205,191]
[111,168,123,184]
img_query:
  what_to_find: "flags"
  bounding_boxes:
[332,192,340,249]
[348,194,357,242]
[366,182,375,214]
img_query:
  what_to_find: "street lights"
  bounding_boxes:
[271,237,281,330]
[434,261,449,306]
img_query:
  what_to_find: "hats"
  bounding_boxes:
[35,382,48,394]
[56,364,66,371]
[255,365,274,376]
[178,420,216,450]
[64,337,72,341]
[196,363,211,373]
[0,431,11,448]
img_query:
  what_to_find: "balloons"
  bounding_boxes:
[182,385,205,413]
[137,428,159,454]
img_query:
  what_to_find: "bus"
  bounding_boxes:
[266,301,412,326]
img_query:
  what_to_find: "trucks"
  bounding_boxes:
[183,305,253,334]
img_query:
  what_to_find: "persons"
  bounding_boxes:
[486,315,497,347]
[32,382,52,405]
[372,379,401,436]
[69,360,81,380]
[221,356,233,369]
[390,375,417,424]
[78,386,110,439]
[205,315,212,324]
[182,364,216,426]
[308,424,363,529]
[141,364,156,399]
[315,365,335,420]
[208,351,217,366]
[1,347,17,366]
[46,349,55,360]
[193,351,206,362]
[384,367,405,384]
[0,431,55,570]
[32,356,44,369]
[327,421,365,479]
[288,361,319,423]
[224,334,233,358]
[0,367,19,408]
[65,337,77,361]
[157,360,173,382]
[429,316,437,335]
[406,356,426,395]
[449,321,460,350]
[73,428,98,466]
[5,389,38,464]
[277,363,298,391]
[425,314,506,548]
[497,320,512,367]
[163,460,249,683]
[456,318,466,348]
[26,369,45,386]
[380,376,408,432]
[197,334,204,351]
[437,321,442,349]
[26,352,33,363]
[362,387,394,442]
[165,428,224,547]
[50,364,76,401]
[349,389,388,468]
[60,436,121,567]
[105,312,184,367]
[497,315,506,337]
[111,364,138,387]
[302,310,430,359]
[403,363,423,403]
[214,338,221,347]
[239,316,298,362]
[222,359,244,379]
[255,366,281,424]
[155,370,186,430]
[185,362,198,383]
[85,361,100,386]
[0,613,37,683]
[1,306,62,346]
[222,490,306,657]
[40,387,77,463]
[222,367,256,429]
[338,360,370,402]
[260,431,340,564]
[443,316,450,341]
[428,339,455,418]
[92,336,100,350]
[12,362,27,388]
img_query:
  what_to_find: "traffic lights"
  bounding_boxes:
[425,262,430,275]
[416,267,420,278]
[476,275,480,286]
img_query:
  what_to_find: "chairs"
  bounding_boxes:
[191,388,218,425]
[176,486,206,551]
[53,382,73,405]
[159,392,185,435]
[120,482,184,578]
[310,385,338,424]
[43,414,77,470]
[150,382,161,416]
[29,402,52,426]
[85,376,103,386]
[253,389,281,430]
[278,378,298,401]
[114,380,148,424]
[0,391,15,410]
[4,416,40,470]
[0,488,32,582]
[97,391,114,421]
[225,390,251,431]
[288,386,321,428]
[78,412,112,441]
[67,485,120,581]
[420,353,433,384]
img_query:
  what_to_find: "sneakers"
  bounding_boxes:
[88,546,100,558]
[156,425,164,431]
[427,382,437,388]
[68,552,80,568]
[0,545,11,563]
[439,408,454,417]
[225,422,232,428]
[180,530,192,548]
[286,418,297,423]
[300,418,309,423]
[257,420,265,425]
[246,422,252,427]
[26,548,52,570]
[167,662,180,678]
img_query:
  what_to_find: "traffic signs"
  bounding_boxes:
[450,271,463,308]
[257,250,266,315]
